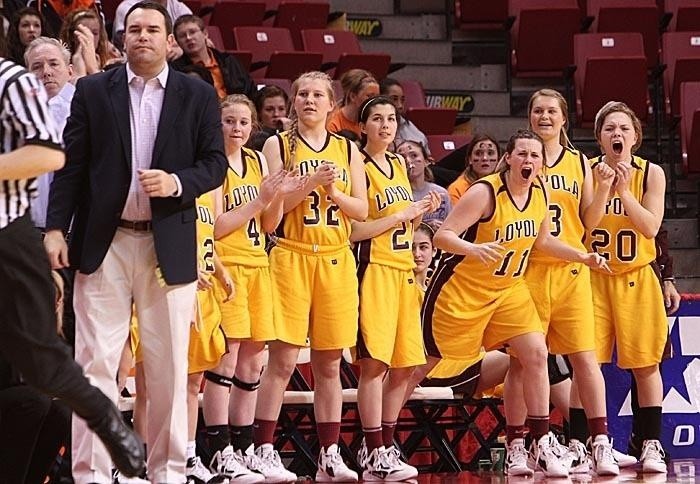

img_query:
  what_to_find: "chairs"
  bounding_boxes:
[184,0,478,164]
[112,338,556,475]
[453,0,699,219]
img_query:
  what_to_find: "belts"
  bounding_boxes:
[117,219,152,231]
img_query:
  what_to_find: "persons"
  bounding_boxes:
[1,0,311,484]
[253,66,682,483]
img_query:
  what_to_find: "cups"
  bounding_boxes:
[491,470,504,484]
[491,447,504,470]
[478,459,490,476]
[478,462,493,480]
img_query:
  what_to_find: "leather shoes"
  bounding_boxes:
[87,400,145,476]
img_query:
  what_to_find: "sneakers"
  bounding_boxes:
[504,434,667,477]
[314,436,419,482]
[186,442,297,483]
